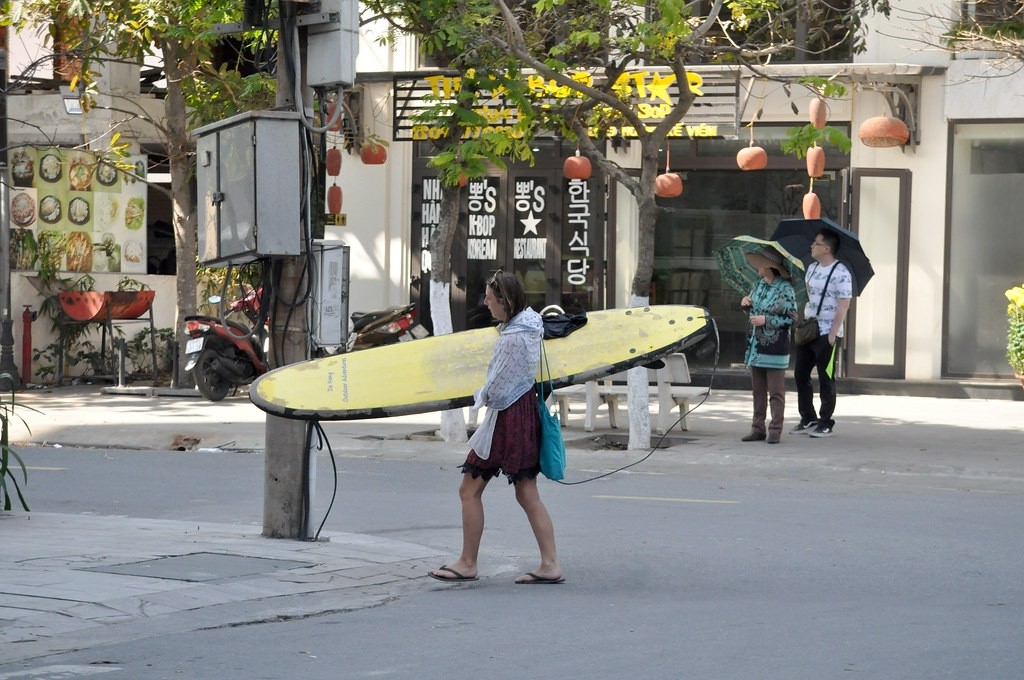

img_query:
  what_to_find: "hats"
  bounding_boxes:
[745,245,791,278]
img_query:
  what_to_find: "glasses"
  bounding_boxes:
[491,269,503,291]
[812,239,826,247]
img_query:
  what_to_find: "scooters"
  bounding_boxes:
[310,303,429,359]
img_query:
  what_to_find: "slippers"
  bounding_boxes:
[428,565,481,581]
[515,572,566,585]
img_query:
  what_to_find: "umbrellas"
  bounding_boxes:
[767,215,875,297]
[715,236,808,310]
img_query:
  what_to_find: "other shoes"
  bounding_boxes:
[742,430,766,441]
[767,430,780,443]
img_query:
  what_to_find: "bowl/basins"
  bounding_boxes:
[12,161,34,183]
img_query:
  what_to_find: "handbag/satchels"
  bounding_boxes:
[755,318,791,356]
[794,316,820,345]
[535,399,566,481]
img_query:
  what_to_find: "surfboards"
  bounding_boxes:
[246,303,713,422]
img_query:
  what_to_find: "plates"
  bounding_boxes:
[13,193,34,223]
[40,154,62,183]
[97,163,118,185]
[69,162,93,188]
[125,198,145,230]
[39,195,61,224]
[123,239,145,268]
[68,197,90,225]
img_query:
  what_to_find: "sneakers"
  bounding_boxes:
[808,425,833,437]
[788,420,819,434]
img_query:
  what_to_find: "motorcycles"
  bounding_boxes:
[183,285,273,402]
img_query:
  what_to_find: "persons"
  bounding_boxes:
[426,267,565,584]
[740,245,798,442]
[790,230,852,437]
